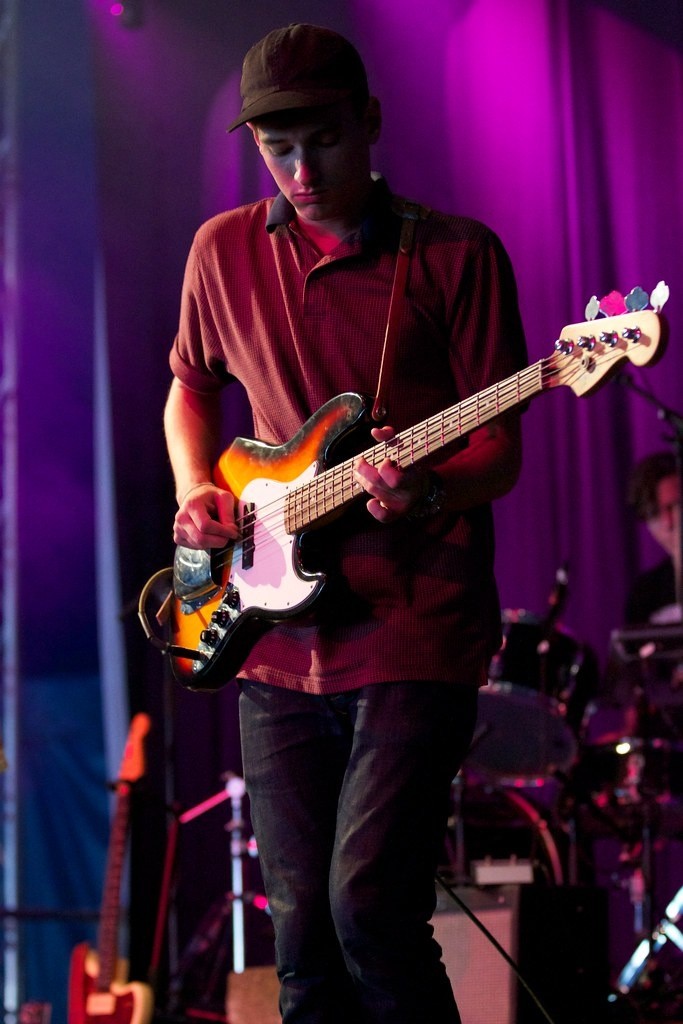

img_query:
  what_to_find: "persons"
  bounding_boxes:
[620,449,682,838]
[161,24,532,1024]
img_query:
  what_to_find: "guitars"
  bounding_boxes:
[166,281,670,692]
[68,714,156,1024]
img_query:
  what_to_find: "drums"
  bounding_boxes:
[462,609,596,787]
[447,776,569,893]
[586,737,678,810]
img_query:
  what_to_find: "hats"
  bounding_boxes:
[226,25,367,133]
